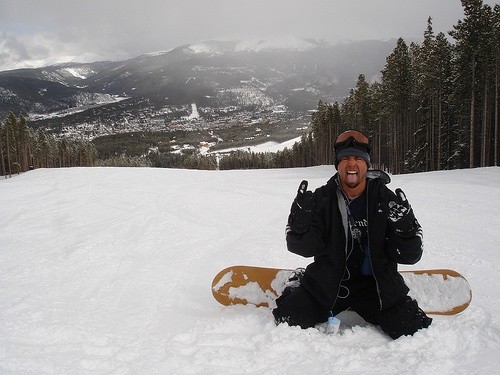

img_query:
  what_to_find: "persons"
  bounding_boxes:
[273,129,433,341]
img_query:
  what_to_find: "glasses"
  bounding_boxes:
[334,130,370,153]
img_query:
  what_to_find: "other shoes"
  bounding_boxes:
[284,267,306,287]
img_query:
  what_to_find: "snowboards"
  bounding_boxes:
[211,265,473,315]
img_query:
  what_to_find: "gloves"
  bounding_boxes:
[376,188,419,239]
[288,180,316,234]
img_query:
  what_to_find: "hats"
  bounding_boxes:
[335,145,371,171]
